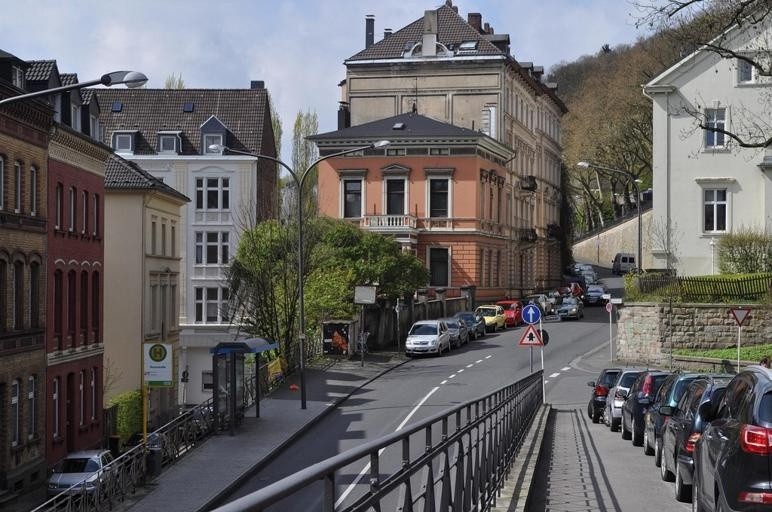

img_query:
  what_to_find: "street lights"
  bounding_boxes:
[2,69,152,107]
[205,138,392,412]
[576,160,643,274]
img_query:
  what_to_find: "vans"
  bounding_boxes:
[611,252,637,274]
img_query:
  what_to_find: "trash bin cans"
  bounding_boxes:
[146,447,162,477]
[108,434,121,458]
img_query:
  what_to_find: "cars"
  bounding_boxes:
[173,405,217,440]
[120,431,170,468]
[405,263,609,356]
[579,356,771,512]
[48,448,120,499]
